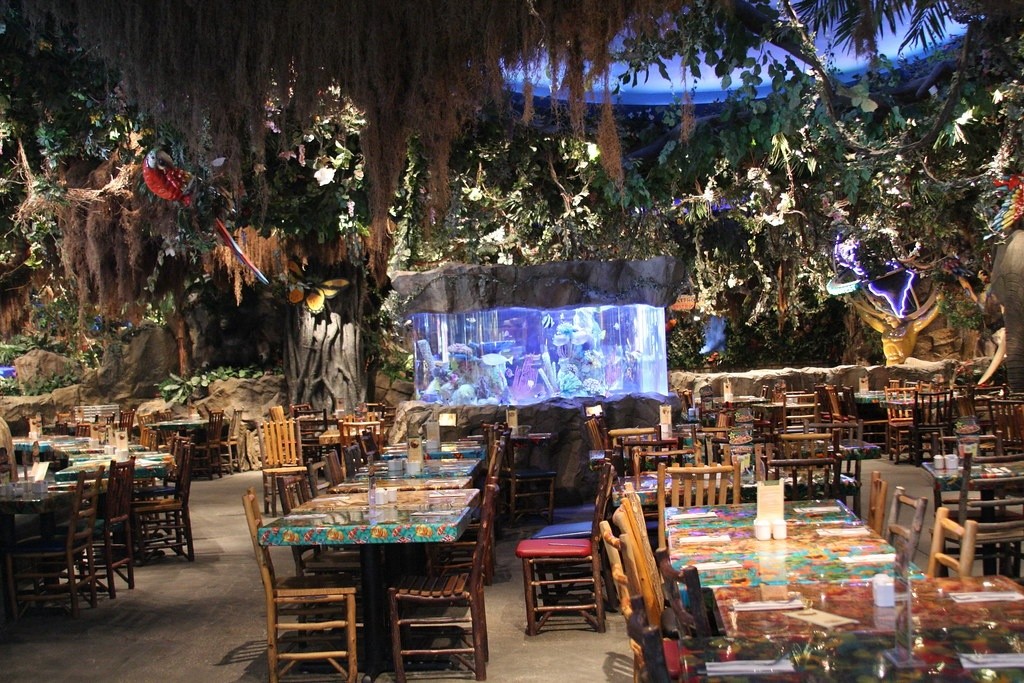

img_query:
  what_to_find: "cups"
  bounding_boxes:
[754,517,787,539]
[724,394,734,402]
[406,462,420,473]
[115,449,128,458]
[934,454,958,469]
[376,487,397,503]
[388,460,402,470]
[873,573,894,607]
[661,424,671,440]
[104,445,112,453]
[426,440,438,449]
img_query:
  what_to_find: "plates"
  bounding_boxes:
[955,426,980,436]
[728,436,752,445]
[734,416,755,422]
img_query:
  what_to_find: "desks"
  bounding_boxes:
[0,390,1023,683]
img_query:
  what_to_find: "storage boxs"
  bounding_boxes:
[413,300,669,404]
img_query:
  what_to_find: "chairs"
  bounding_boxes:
[0,375,385,682]
[386,376,1024,683]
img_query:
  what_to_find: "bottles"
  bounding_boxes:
[959,442,977,458]
[732,454,751,471]
